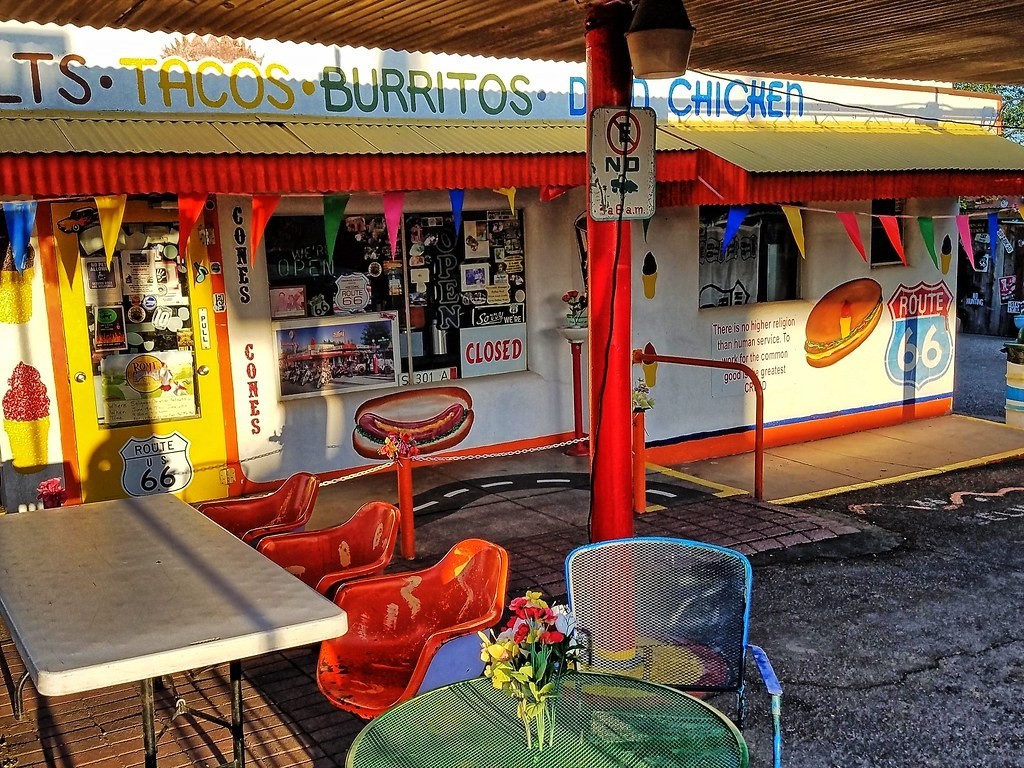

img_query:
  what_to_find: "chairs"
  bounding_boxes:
[256,500,403,601]
[562,537,783,768]
[194,472,320,549]
[315,538,511,720]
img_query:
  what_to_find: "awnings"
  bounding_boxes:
[0,117,700,196]
[656,125,1024,207]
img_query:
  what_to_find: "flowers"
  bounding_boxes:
[474,593,580,748]
[561,289,587,330]
[377,431,420,463]
[632,379,656,412]
[35,478,71,508]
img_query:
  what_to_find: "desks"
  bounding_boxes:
[0,493,351,768]
[342,669,754,768]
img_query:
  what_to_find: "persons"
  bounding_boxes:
[364,354,372,371]
[347,357,354,377]
